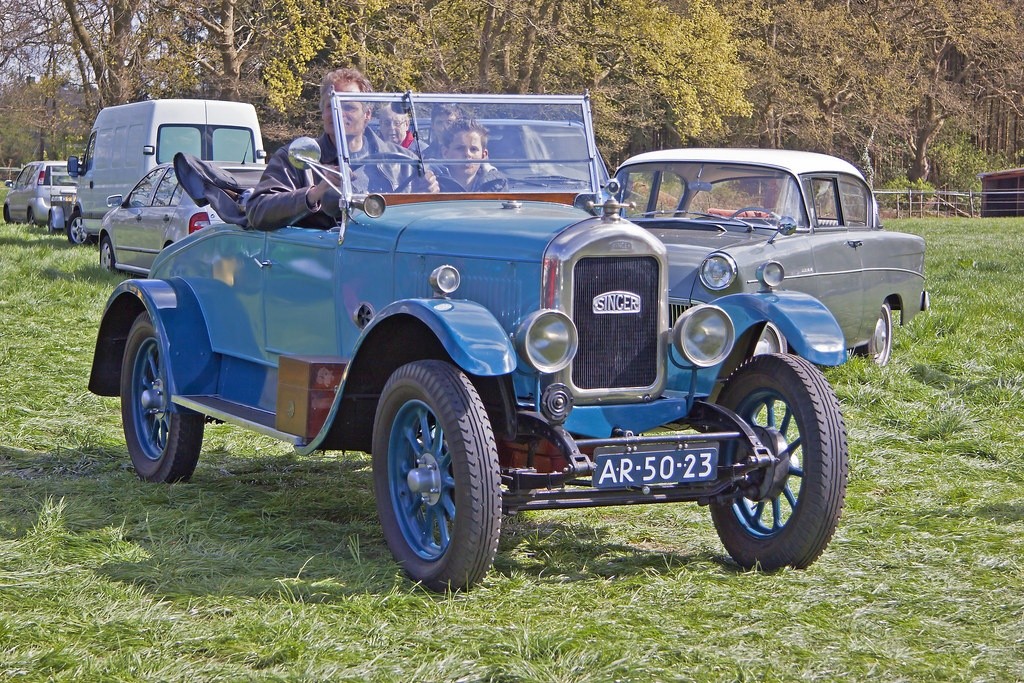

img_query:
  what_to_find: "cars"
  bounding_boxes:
[3,161,80,226]
[608,147,930,367]
[99,161,269,278]
[87,85,851,596]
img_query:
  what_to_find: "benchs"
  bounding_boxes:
[202,169,264,223]
[740,219,839,227]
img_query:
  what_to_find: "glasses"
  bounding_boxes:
[380,119,405,126]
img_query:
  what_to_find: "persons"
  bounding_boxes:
[421,103,462,162]
[246,69,441,230]
[429,116,509,194]
[378,101,429,155]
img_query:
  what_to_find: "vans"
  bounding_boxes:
[67,99,266,244]
